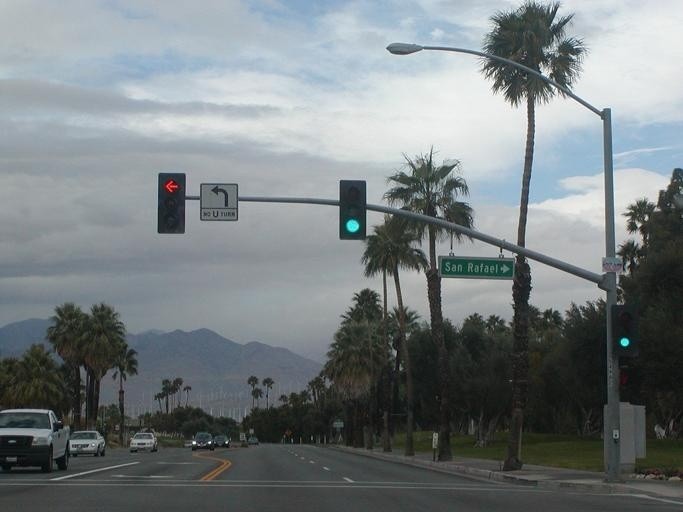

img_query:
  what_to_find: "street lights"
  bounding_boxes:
[385,40,623,486]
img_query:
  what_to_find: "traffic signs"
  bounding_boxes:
[199,182,239,222]
[437,255,516,280]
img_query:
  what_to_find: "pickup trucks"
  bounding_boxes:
[0,407,74,475]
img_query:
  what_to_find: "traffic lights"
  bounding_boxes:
[156,172,187,235]
[337,179,368,241]
[610,303,640,358]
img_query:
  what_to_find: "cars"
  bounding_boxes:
[129,432,159,453]
[68,430,106,457]
[182,431,260,451]
